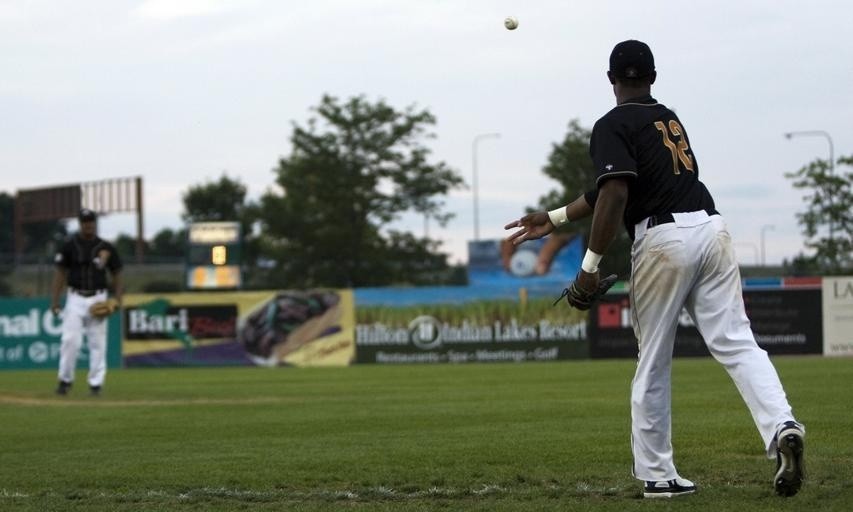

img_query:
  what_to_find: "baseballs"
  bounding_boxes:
[507,18,517,29]
[511,250,539,276]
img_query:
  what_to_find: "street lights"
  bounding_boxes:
[782,128,837,268]
[468,129,507,239]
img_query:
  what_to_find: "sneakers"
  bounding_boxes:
[774,420,804,498]
[644,477,695,499]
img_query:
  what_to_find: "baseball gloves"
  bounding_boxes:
[90,298,117,319]
[566,274,618,310]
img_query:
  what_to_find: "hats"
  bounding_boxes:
[610,40,654,80]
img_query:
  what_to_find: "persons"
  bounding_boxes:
[51,205,122,396]
[503,40,807,500]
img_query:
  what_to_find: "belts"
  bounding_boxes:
[77,290,97,297]
[646,212,673,228]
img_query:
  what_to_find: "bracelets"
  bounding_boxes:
[580,247,602,277]
[546,204,571,226]
